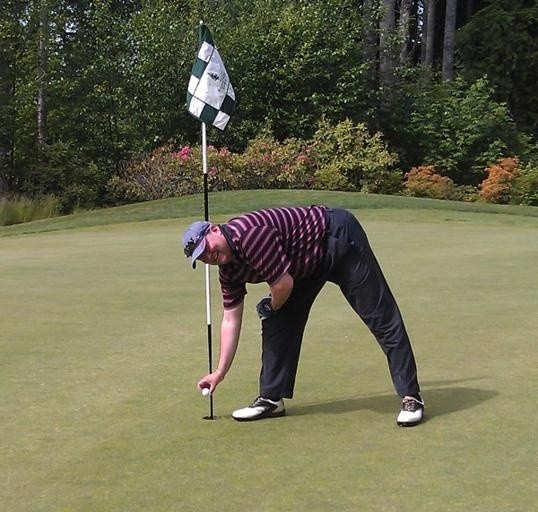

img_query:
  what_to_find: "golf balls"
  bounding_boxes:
[202,388,210,396]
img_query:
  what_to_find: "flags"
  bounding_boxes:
[186,24,236,132]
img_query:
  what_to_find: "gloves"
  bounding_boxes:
[256,297,275,321]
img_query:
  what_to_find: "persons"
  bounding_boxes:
[181,204,424,427]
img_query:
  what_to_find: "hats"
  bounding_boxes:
[182,220,210,269]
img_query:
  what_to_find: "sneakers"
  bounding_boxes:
[231,396,285,421]
[396,395,425,427]
